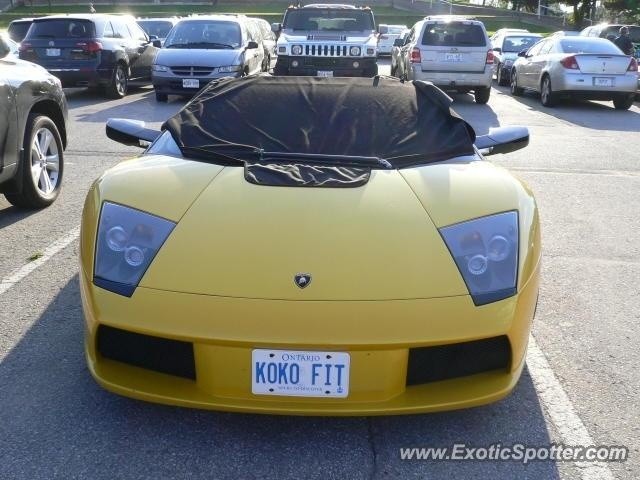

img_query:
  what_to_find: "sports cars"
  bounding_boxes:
[78,75,544,419]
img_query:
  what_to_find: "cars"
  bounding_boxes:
[1,34,70,213]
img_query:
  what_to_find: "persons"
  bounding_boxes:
[616,27,636,56]
[608,35,617,44]
[87,2,96,13]
